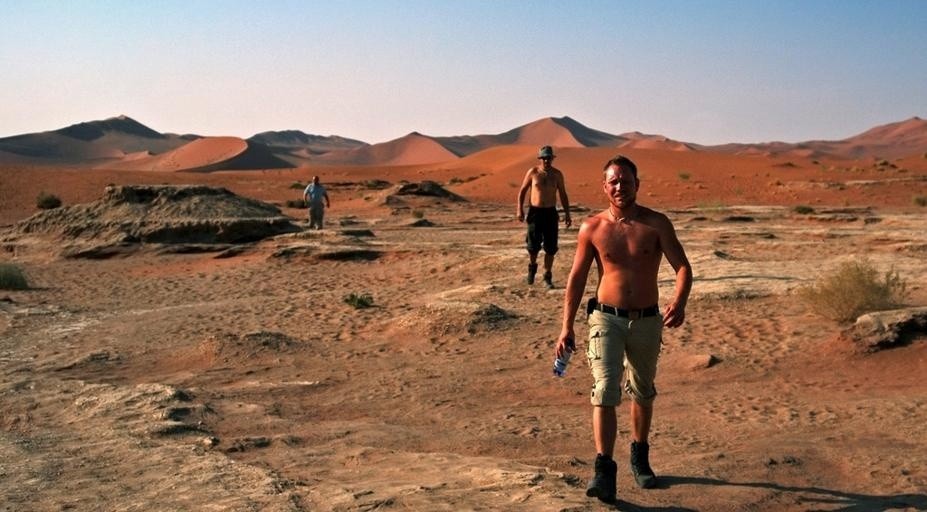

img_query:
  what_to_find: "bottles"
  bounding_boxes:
[553,341,573,377]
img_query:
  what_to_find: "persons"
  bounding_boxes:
[517,145,571,289]
[554,156,694,499]
[304,175,330,229]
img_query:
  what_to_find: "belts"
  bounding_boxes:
[589,302,659,320]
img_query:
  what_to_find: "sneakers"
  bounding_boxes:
[586,452,617,505]
[628,441,657,489]
[526,263,538,284]
[543,270,554,288]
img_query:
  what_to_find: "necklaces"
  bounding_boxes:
[608,203,640,221]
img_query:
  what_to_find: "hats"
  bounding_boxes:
[537,145,557,159]
